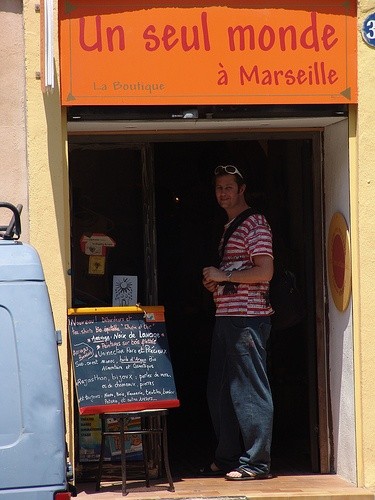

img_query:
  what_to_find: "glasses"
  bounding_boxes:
[215,165,243,180]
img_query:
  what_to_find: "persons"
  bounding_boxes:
[191,163,274,480]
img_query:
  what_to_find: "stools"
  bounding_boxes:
[95,409,175,497]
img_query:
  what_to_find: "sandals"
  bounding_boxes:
[198,460,238,475]
[225,468,271,480]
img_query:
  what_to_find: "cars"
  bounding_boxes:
[0,198,76,500]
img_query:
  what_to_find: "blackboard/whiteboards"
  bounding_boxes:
[67,305,180,416]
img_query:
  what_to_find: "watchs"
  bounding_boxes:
[225,270,231,278]
